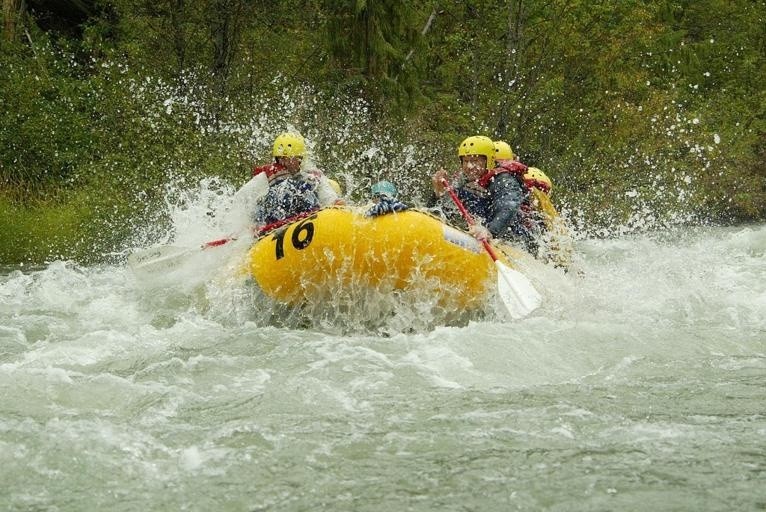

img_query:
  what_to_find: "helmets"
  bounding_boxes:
[457,135,495,172]
[370,180,398,200]
[521,166,552,192]
[271,132,306,157]
[326,178,341,195]
[491,141,514,166]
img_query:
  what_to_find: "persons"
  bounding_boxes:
[328,179,341,199]
[426,136,544,261]
[521,165,552,197]
[371,181,397,204]
[232,134,346,250]
[493,140,573,273]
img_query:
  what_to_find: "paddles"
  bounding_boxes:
[128,213,313,281]
[441,178,540,319]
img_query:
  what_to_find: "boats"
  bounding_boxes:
[230,203,575,329]
